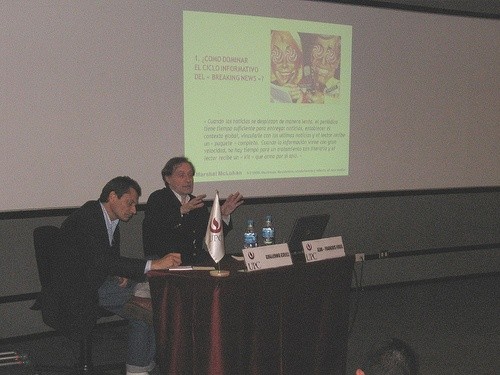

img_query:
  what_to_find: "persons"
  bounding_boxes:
[31,176,183,374]
[355,337,417,375]
[142,156,245,265]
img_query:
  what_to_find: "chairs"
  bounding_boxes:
[31,225,127,375]
[143,217,167,312]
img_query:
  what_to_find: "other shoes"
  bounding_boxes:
[123,296,153,321]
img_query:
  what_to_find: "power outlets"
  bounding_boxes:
[355,254,365,262]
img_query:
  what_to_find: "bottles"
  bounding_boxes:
[262,216,274,245]
[243,220,257,248]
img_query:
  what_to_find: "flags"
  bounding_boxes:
[202,192,226,263]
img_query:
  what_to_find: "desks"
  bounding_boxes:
[147,253,353,375]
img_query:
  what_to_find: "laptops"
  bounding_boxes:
[288,213,330,255]
